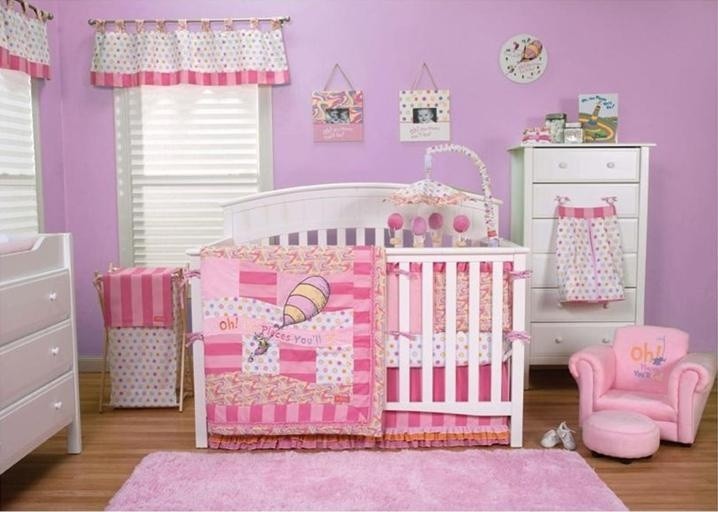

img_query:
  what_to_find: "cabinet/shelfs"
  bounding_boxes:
[588,106,600,126]
[545,114,566,144]
[564,123,587,144]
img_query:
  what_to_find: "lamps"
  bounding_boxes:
[566,321,715,449]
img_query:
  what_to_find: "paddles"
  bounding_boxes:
[102,444,630,509]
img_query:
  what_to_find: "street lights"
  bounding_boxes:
[312,89,364,143]
[399,88,451,142]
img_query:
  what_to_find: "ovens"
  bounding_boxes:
[187,179,528,450]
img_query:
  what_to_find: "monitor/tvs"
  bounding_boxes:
[578,92,619,144]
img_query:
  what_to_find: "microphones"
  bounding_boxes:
[581,410,661,463]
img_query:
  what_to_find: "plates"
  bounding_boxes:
[512,140,649,392]
[94,264,185,414]
[0,228,84,480]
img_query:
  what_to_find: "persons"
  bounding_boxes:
[325,109,349,124]
[415,108,435,124]
